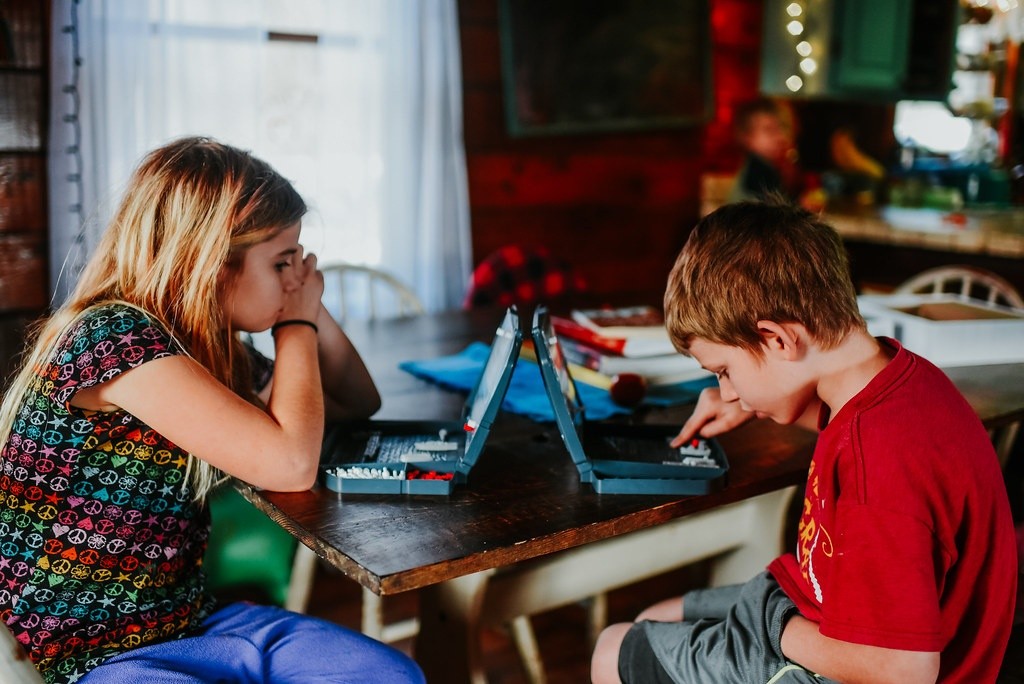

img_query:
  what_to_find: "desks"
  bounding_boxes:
[233,295,1024,684]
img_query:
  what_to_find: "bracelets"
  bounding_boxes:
[271,319,318,336]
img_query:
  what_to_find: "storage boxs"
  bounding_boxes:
[532,301,736,497]
[328,300,524,496]
[857,295,1024,369]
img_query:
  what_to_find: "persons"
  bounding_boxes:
[589,198,1019,684]
[734,93,884,214]
[0,135,425,684]
